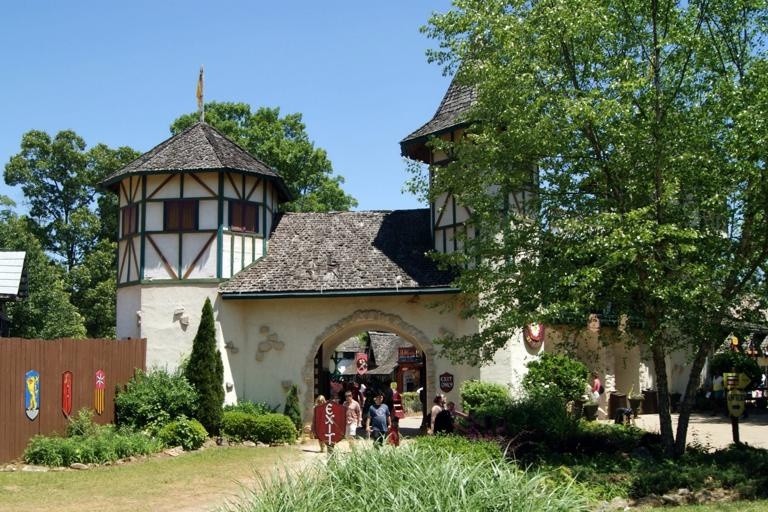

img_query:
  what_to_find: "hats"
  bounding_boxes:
[373,388,384,398]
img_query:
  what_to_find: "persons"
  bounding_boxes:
[591,371,608,420]
[311,378,457,453]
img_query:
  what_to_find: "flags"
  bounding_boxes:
[196,75,204,114]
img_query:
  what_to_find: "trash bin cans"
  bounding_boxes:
[609,391,627,419]
[642,388,658,414]
